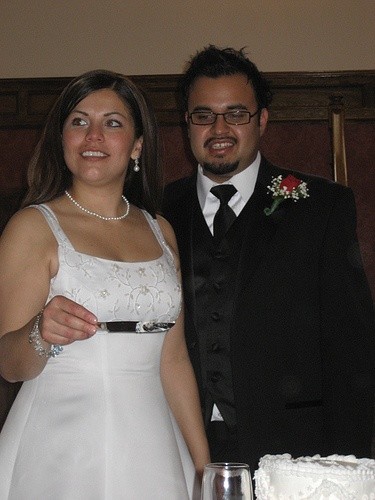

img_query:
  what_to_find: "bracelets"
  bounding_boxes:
[28,308,64,359]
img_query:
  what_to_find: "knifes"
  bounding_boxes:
[97,320,176,333]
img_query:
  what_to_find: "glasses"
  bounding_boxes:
[187,111,258,126]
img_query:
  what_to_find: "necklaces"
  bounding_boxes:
[64,190,130,221]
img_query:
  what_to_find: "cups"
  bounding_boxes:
[199,462,254,500]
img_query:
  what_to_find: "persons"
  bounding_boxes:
[0,69,212,500]
[152,44,375,500]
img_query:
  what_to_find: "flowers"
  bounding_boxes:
[263,174,310,217]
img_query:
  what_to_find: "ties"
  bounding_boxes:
[209,184,238,243]
